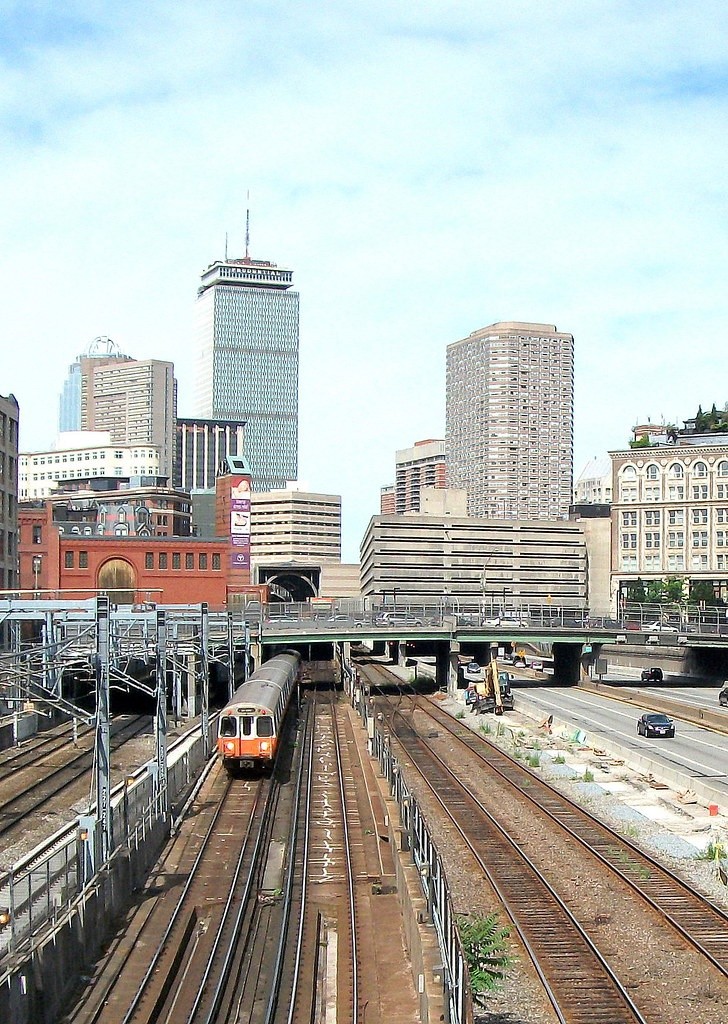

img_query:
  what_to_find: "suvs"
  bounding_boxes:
[267,615,297,624]
[376,612,422,627]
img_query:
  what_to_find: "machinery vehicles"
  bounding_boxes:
[467,654,514,715]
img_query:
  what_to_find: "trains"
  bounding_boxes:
[217,648,301,776]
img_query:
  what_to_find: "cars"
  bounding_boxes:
[328,616,363,628]
[546,618,584,628]
[636,714,676,738]
[593,619,623,629]
[483,616,529,627]
[468,663,480,673]
[642,621,678,633]
[621,622,640,629]
[718,687,728,708]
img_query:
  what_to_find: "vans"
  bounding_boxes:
[641,667,664,681]
[531,660,543,669]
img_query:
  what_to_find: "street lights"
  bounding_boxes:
[364,598,368,612]
[33,555,41,589]
[394,587,400,611]
[504,587,510,616]
